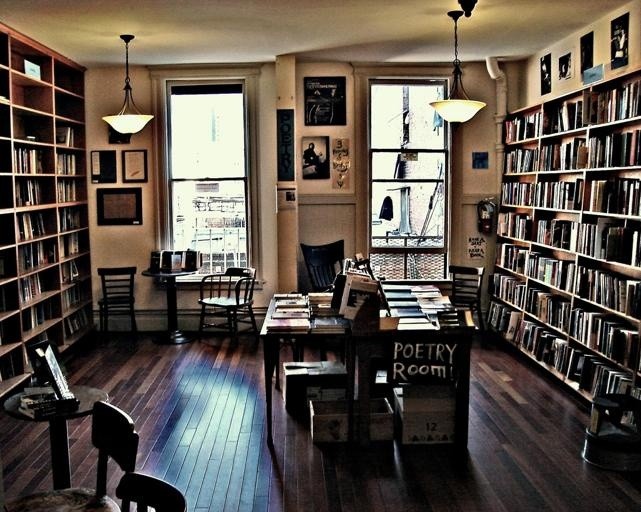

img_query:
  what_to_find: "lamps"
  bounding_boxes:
[101,34,157,134]
[428,10,488,125]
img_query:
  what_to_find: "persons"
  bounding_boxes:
[614,27,627,58]
[303,141,323,166]
[334,140,348,155]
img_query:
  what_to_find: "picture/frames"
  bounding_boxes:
[303,75,347,127]
[122,150,148,183]
[96,186,144,227]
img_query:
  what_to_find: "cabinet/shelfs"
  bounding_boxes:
[487,103,543,356]
[564,67,641,443]
[9,27,64,378]
[518,84,586,384]
[54,51,98,353]
[0,23,28,398]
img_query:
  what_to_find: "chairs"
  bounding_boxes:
[96,266,139,345]
[445,263,487,351]
[197,266,261,350]
[0,399,142,512]
[298,239,348,291]
[114,471,189,512]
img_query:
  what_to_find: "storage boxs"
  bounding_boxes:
[392,384,461,446]
[308,397,394,442]
[280,360,350,417]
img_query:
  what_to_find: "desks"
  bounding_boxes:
[2,385,116,492]
[258,284,353,451]
[353,280,483,451]
[139,267,200,347]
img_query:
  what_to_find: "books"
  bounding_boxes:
[497,178,640,268]
[151,249,204,272]
[504,79,641,173]
[1,120,100,422]
[488,243,641,412]
[259,252,475,333]
